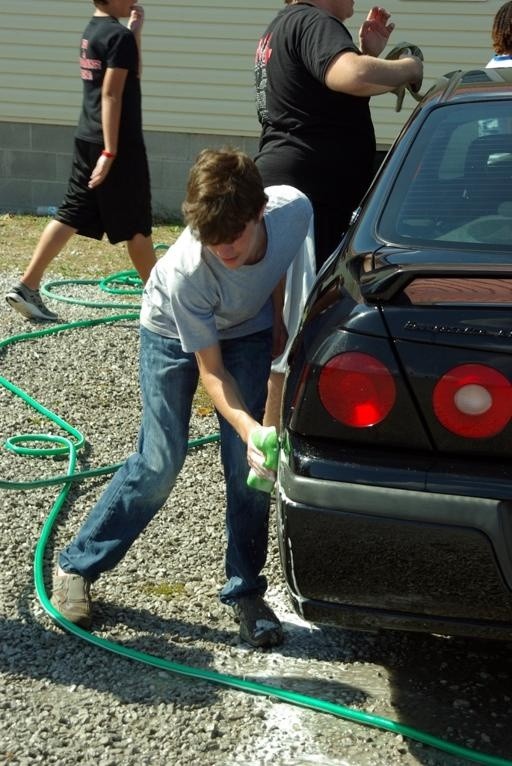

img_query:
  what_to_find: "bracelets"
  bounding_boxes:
[101,149,118,159]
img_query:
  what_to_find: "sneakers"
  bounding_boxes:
[233,595,283,648]
[4,280,59,321]
[51,568,93,630]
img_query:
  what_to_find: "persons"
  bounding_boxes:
[47,144,321,651]
[3,0,159,322]
[474,0,511,166]
[251,0,425,278]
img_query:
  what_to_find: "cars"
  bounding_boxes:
[274,66,510,641]
